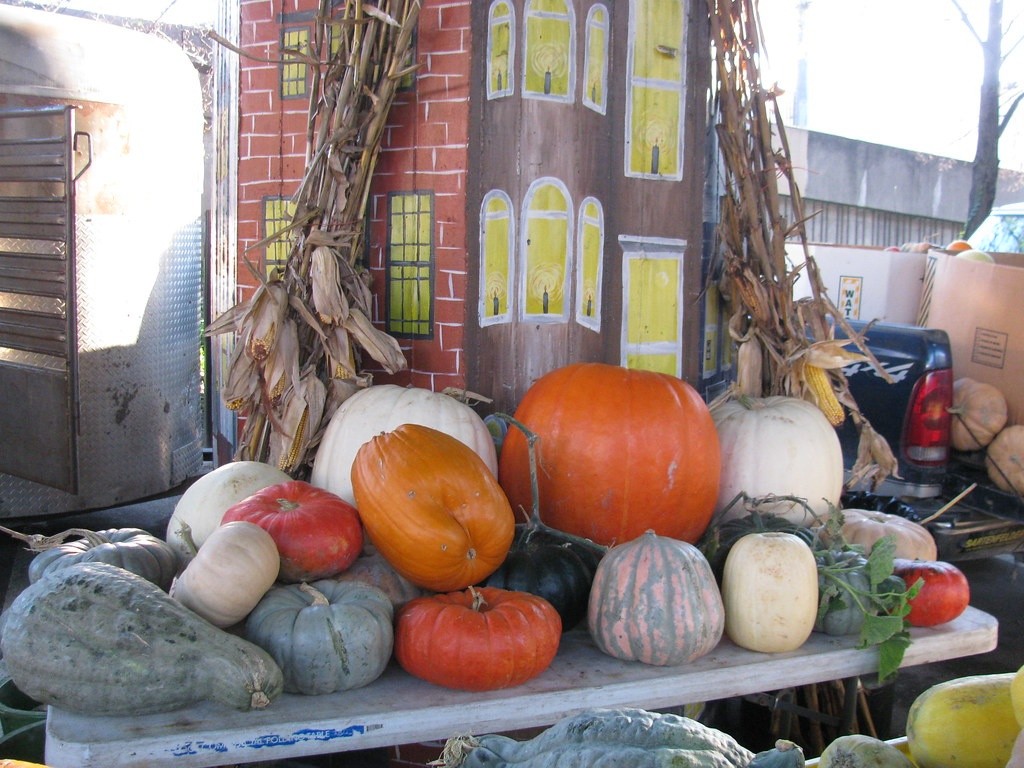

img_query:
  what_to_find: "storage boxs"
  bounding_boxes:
[782,241,928,327]
[916,248,1024,467]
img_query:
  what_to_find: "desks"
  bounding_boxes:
[45,603,997,768]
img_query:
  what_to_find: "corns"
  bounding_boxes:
[223,246,406,473]
[803,360,846,426]
[737,334,763,397]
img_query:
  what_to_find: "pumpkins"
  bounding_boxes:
[0,528,179,585]
[426,665,1024,768]
[0,561,283,717]
[985,424,1024,494]
[944,377,1007,451]
[351,424,515,591]
[311,384,499,510]
[588,529,725,667]
[394,585,562,690]
[169,461,422,628]
[696,490,970,653]
[499,362,722,545]
[710,395,843,528]
[480,529,600,634]
[247,580,395,696]
